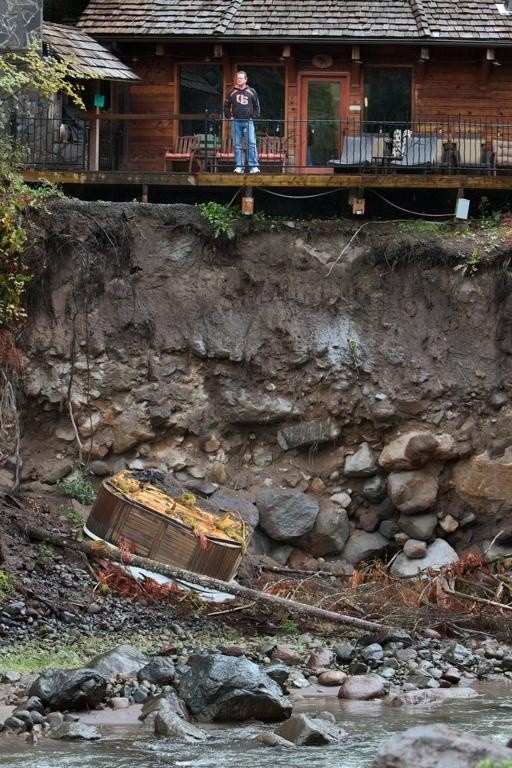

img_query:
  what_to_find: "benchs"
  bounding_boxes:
[210,132,289,177]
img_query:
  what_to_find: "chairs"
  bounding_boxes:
[164,135,203,174]
[389,134,439,174]
[491,138,511,176]
[326,134,373,174]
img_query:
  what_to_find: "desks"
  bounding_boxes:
[370,155,403,175]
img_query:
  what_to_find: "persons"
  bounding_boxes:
[224,70,262,173]
[307,122,314,167]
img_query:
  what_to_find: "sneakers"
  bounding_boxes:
[249,167,261,174]
[233,167,246,174]
